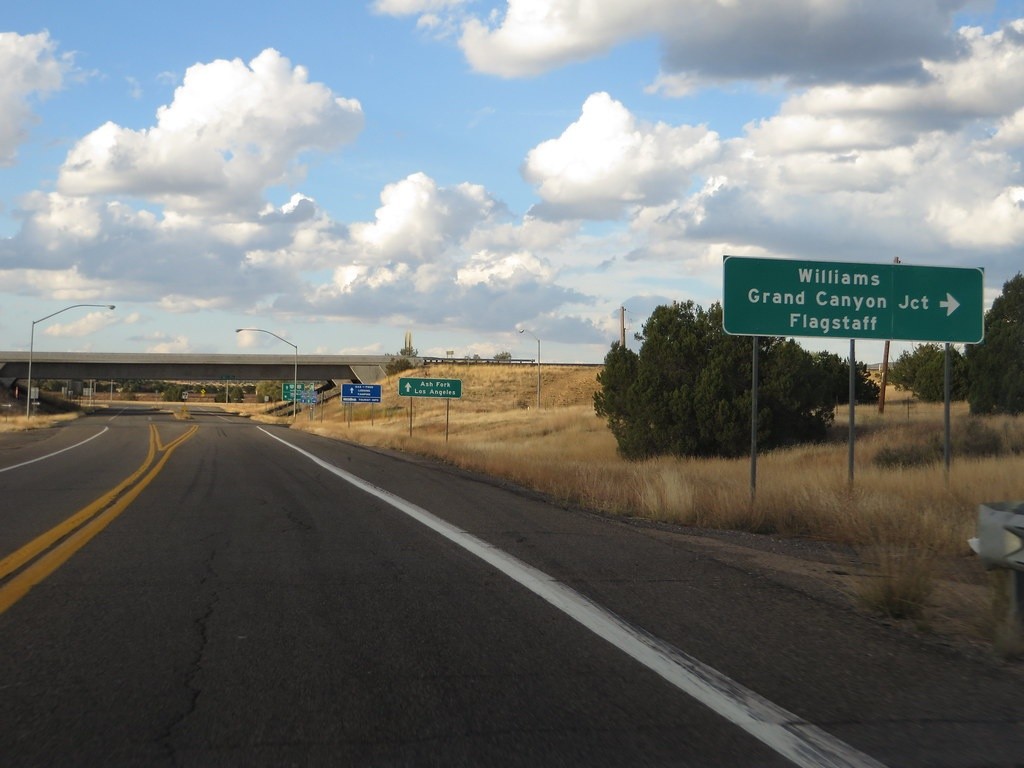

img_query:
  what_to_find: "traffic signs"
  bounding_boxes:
[282,383,305,401]
[722,255,985,343]
[341,384,382,403]
[301,391,317,404]
[398,378,462,399]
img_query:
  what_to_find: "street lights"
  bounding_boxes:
[235,328,297,419]
[27,304,115,419]
